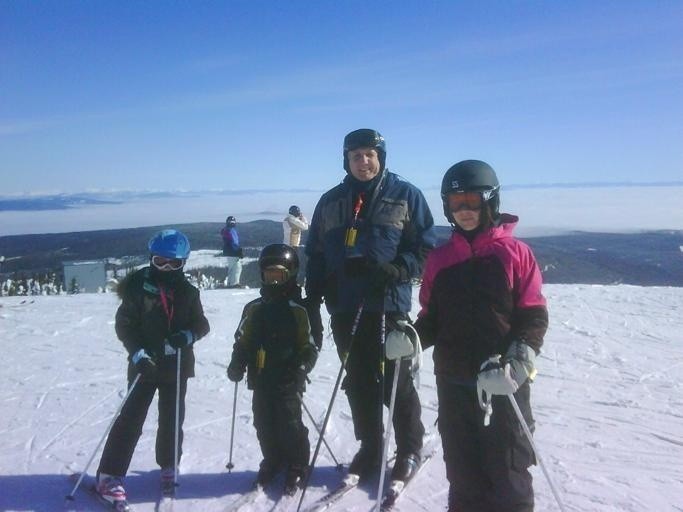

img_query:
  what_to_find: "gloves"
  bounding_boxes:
[131,346,160,382]
[478,340,536,395]
[385,326,423,361]
[380,258,409,287]
[169,329,193,349]
[306,276,326,305]
[226,359,245,381]
[284,367,308,392]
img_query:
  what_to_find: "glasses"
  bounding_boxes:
[152,256,186,269]
[446,188,498,211]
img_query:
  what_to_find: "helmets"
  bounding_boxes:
[343,130,387,170]
[290,206,300,217]
[226,217,235,225]
[148,230,190,260]
[260,244,301,303]
[441,160,500,222]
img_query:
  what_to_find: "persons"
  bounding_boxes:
[221,216,246,289]
[228,245,324,487]
[283,206,309,246]
[384,160,548,512]
[304,128,434,480]
[94,229,209,503]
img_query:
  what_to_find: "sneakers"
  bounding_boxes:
[96,470,126,502]
[159,464,178,483]
[257,458,277,481]
[349,445,376,476]
[287,460,307,486]
[392,448,421,479]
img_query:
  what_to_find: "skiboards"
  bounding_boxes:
[301,451,432,512]
[71,468,180,512]
[222,463,308,512]
